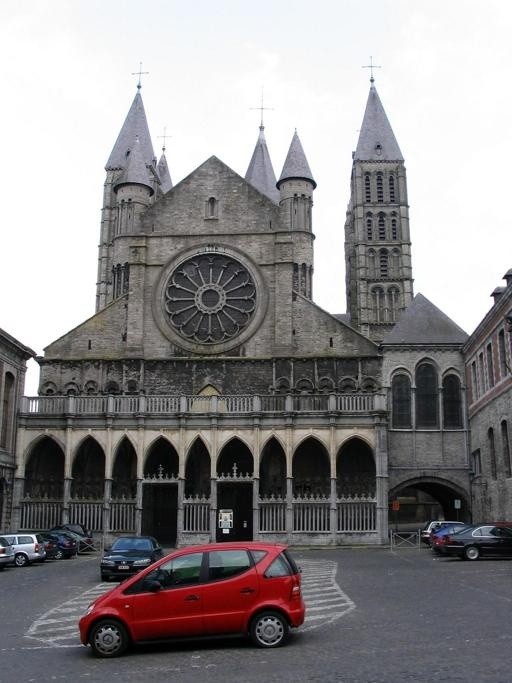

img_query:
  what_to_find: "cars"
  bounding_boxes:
[422,519,511,560]
[0,523,92,566]
[79,541,306,657]
[102,534,163,577]
